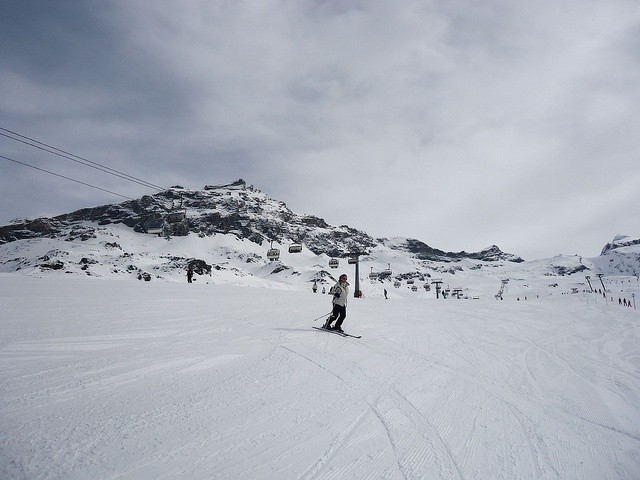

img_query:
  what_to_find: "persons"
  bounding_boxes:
[311,281,317,293]
[186,266,193,284]
[517,286,633,309]
[322,287,326,294]
[267,251,279,261]
[357,290,362,299]
[383,288,387,300]
[322,274,349,334]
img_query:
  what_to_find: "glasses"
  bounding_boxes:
[341,278,347,280]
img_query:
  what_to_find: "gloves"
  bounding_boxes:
[335,293,340,298]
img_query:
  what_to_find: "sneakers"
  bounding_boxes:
[324,324,331,330]
[335,327,344,332]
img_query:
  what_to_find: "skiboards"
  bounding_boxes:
[314,326,362,339]
